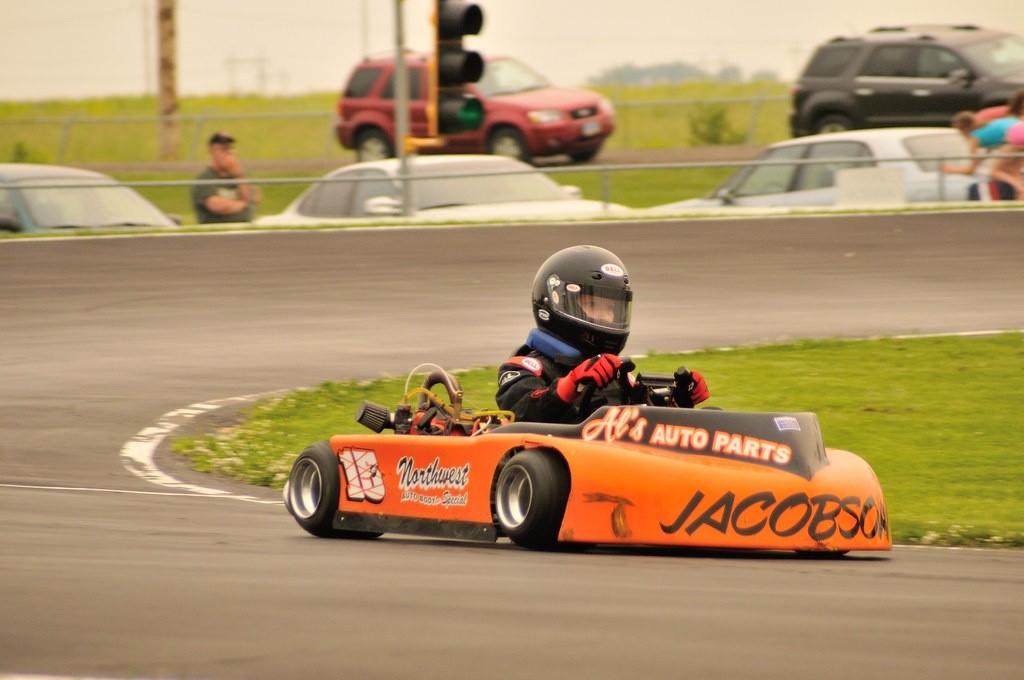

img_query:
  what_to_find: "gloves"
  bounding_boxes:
[557,353,621,404]
[671,370,709,408]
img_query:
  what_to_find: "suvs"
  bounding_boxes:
[335,51,617,165]
[788,22,1023,140]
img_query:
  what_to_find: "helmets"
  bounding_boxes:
[531,244,633,359]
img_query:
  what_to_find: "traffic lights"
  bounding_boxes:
[429,0,484,136]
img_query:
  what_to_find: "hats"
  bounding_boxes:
[209,133,235,143]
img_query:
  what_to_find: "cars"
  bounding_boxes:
[647,125,977,218]
[254,154,652,228]
[0,163,186,233]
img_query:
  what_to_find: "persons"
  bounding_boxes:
[495,246,713,424]
[190,133,262,224]
[943,89,1024,202]
